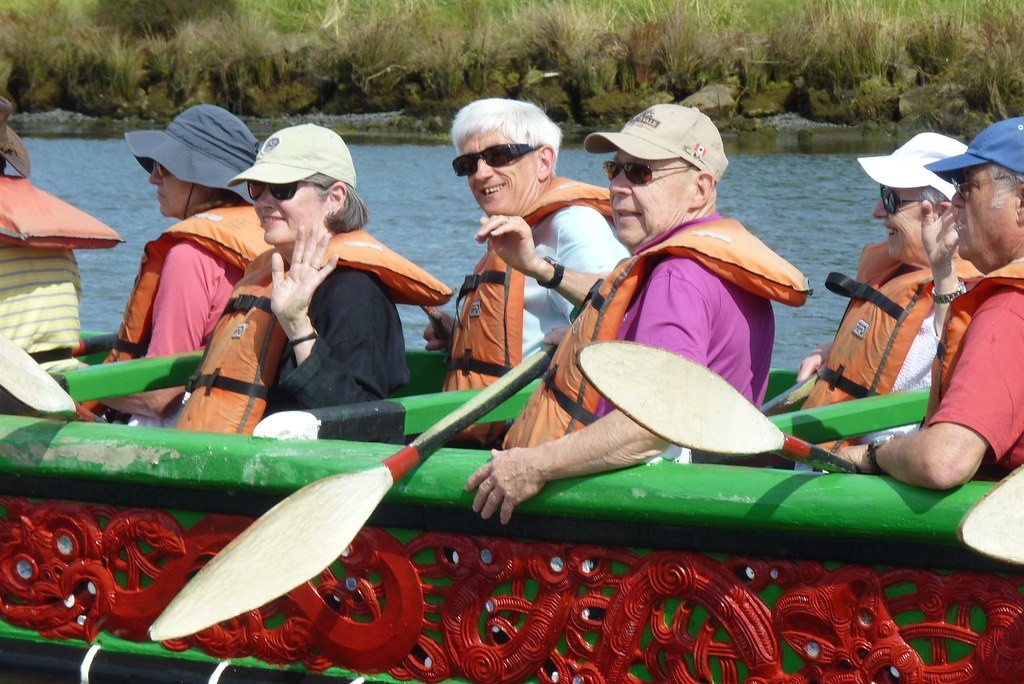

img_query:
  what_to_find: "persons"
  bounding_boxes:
[738,132,985,472]
[462,104,775,526]
[823,115,1024,490]
[72,104,275,429]
[0,98,82,421]
[226,122,412,419]
[423,97,631,450]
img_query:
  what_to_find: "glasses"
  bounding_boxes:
[152,162,168,177]
[950,175,1024,201]
[452,144,542,177]
[879,183,922,214]
[602,160,693,184]
[247,181,328,200]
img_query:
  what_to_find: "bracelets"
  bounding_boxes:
[289,327,319,346]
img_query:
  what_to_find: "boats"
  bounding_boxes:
[0,324,1024,684]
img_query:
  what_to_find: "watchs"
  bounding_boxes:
[868,432,897,477]
[929,281,967,303]
[536,256,565,290]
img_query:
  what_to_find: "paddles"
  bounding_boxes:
[763,372,820,416]
[957,465,1024,567]
[72,333,117,356]
[0,335,109,423]
[421,305,451,343]
[573,337,857,475]
[146,343,560,642]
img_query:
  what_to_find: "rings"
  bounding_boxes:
[311,263,320,271]
[506,217,510,221]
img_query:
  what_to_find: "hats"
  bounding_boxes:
[857,132,969,201]
[924,117,1024,185]
[227,124,356,189]
[124,105,262,207]
[584,103,729,183]
[0,97,31,177]
